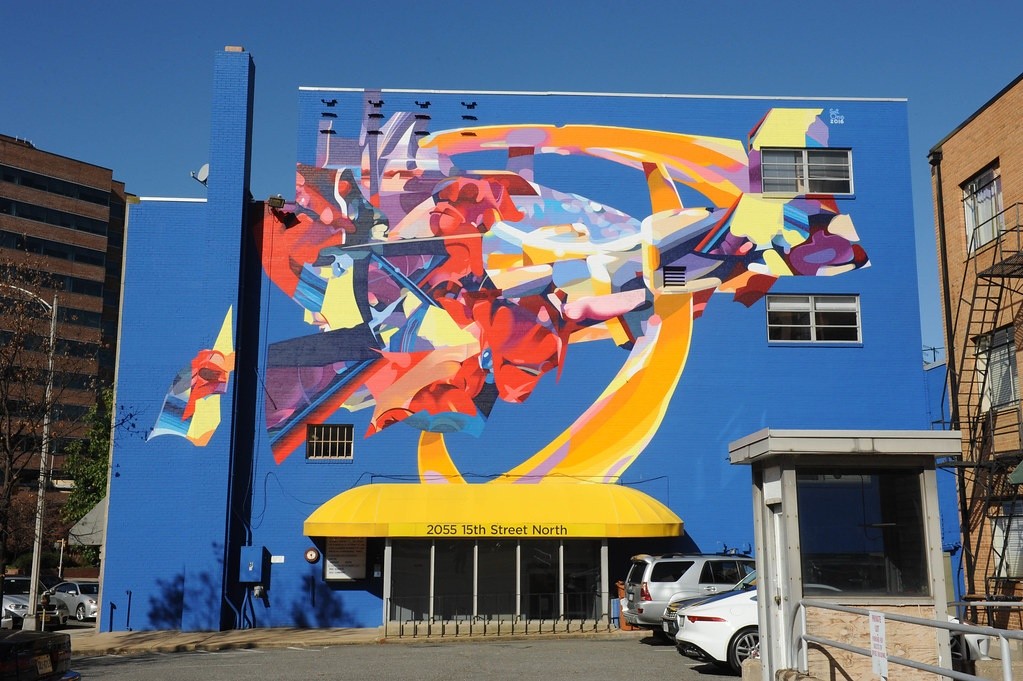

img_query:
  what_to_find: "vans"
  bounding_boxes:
[624,553,756,642]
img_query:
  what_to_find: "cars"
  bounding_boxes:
[48,582,99,622]
[1,576,69,631]
[661,569,761,679]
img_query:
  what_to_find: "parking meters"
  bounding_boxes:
[41,591,50,632]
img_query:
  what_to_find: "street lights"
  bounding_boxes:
[57,538,67,578]
